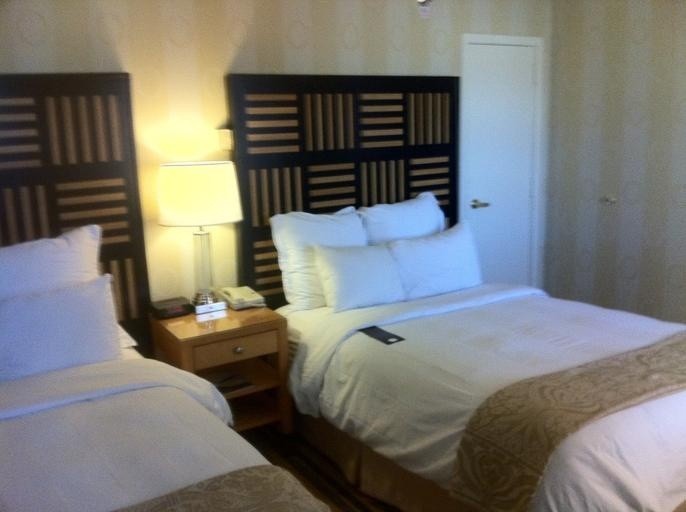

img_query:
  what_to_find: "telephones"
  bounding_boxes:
[218,286,265,310]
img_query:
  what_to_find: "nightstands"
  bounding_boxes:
[150,293,294,441]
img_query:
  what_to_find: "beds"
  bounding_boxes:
[228,74,685,512]
[1,74,327,511]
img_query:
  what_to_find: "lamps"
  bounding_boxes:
[155,159,244,302]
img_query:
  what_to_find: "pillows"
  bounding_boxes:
[269,208,373,309]
[2,224,135,385]
[388,222,484,303]
[310,239,402,309]
[356,192,449,244]
[1,274,124,386]
